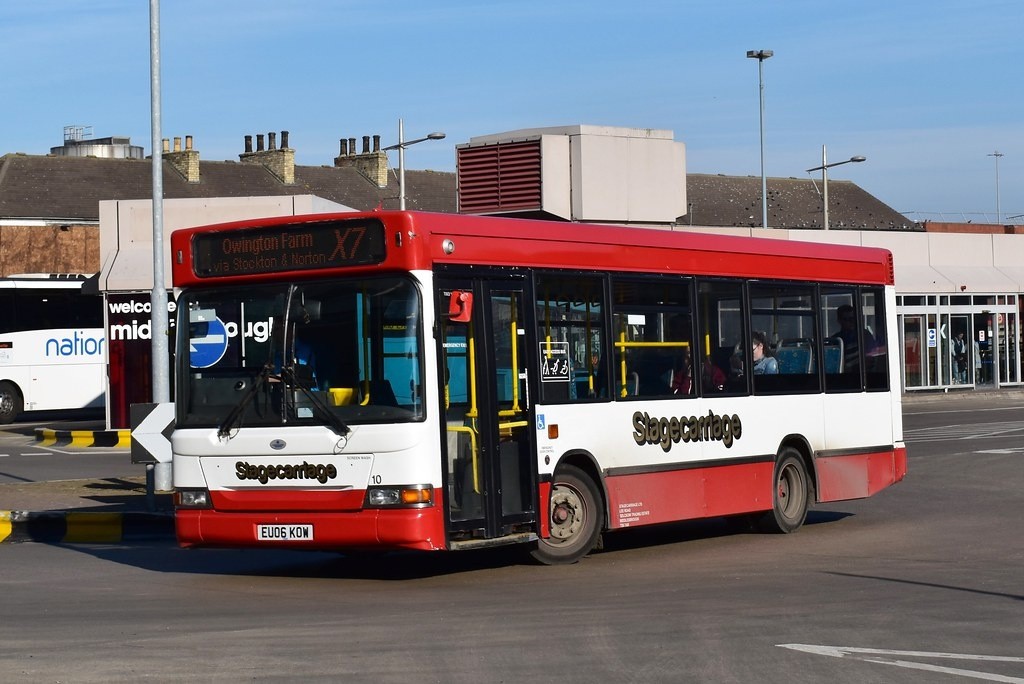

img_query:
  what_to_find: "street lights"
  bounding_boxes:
[746,49,775,229]
[381,118,446,210]
[805,144,867,230]
[993,150,1005,224]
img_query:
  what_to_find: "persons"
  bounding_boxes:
[951,330,981,385]
[671,331,780,394]
[828,304,876,375]
[262,323,321,393]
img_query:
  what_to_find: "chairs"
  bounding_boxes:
[774,337,814,374]
[822,337,844,373]
[610,372,643,399]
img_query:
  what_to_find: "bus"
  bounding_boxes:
[170,210,906,567]
[0,272,107,424]
